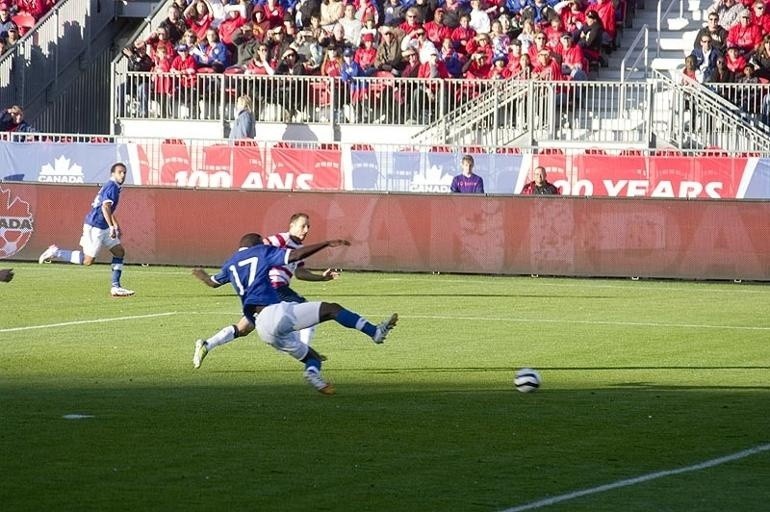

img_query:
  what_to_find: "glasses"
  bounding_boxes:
[159,3,554,64]
[701,0,770,65]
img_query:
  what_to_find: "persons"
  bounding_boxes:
[449,154,483,193]
[0,104,45,142]
[192,213,340,370]
[0,268,13,284]
[191,233,398,396]
[0,0,59,56]
[38,163,134,297]
[228,95,256,139]
[680,1,770,132]
[519,166,558,196]
[121,0,623,124]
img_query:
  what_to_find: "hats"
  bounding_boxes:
[587,11,598,20]
[560,32,572,40]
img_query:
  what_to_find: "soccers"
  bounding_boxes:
[514,368,539,393]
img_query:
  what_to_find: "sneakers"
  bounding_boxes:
[111,287,134,298]
[373,314,399,345]
[193,341,208,370]
[304,372,337,395]
[38,243,58,265]
